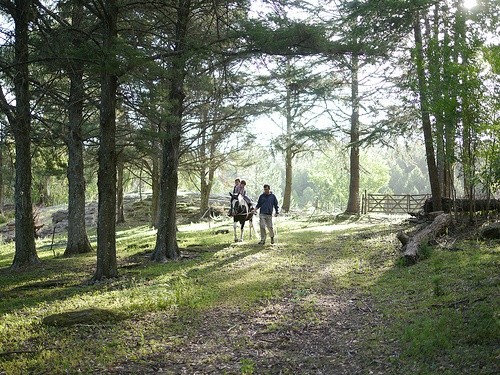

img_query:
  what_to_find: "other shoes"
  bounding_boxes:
[271,237,274,244]
[258,242,265,244]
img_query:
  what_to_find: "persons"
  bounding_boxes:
[235,180,252,206]
[230,179,254,208]
[251,185,279,245]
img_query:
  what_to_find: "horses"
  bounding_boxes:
[229,191,260,242]
